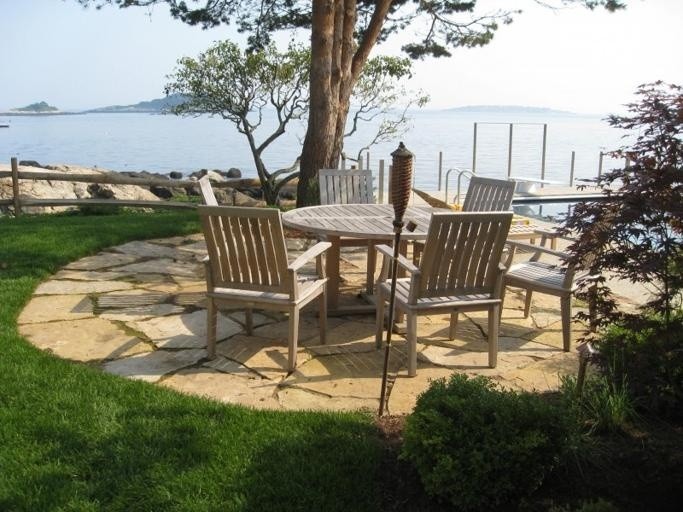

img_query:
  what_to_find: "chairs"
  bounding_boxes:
[198,175,227,247]
[316,169,374,279]
[497,238,597,353]
[461,176,517,211]
[375,210,514,378]
[198,205,334,374]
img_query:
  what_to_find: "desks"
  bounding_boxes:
[508,223,563,250]
[281,203,502,335]
